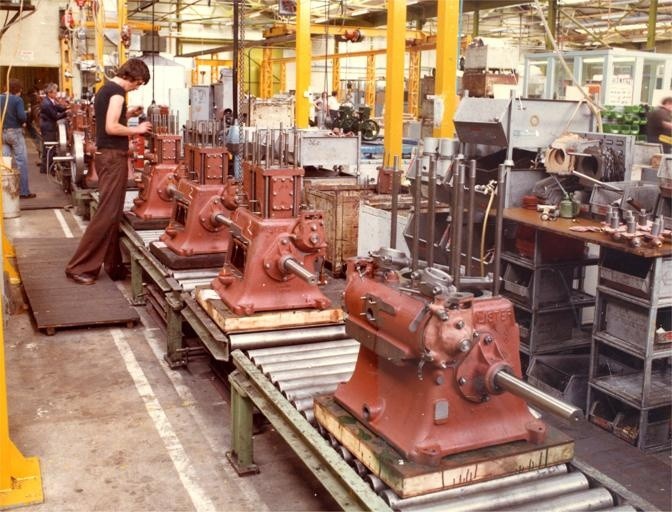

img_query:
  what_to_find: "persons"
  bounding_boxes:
[1,78,37,199]
[646,96,672,154]
[327,90,339,123]
[65,58,153,285]
[313,91,327,120]
[39,83,72,174]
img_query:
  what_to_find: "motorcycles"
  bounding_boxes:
[335,103,379,141]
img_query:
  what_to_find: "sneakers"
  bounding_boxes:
[20,193,36,198]
[65,271,96,286]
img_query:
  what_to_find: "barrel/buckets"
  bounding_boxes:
[2,169,21,218]
